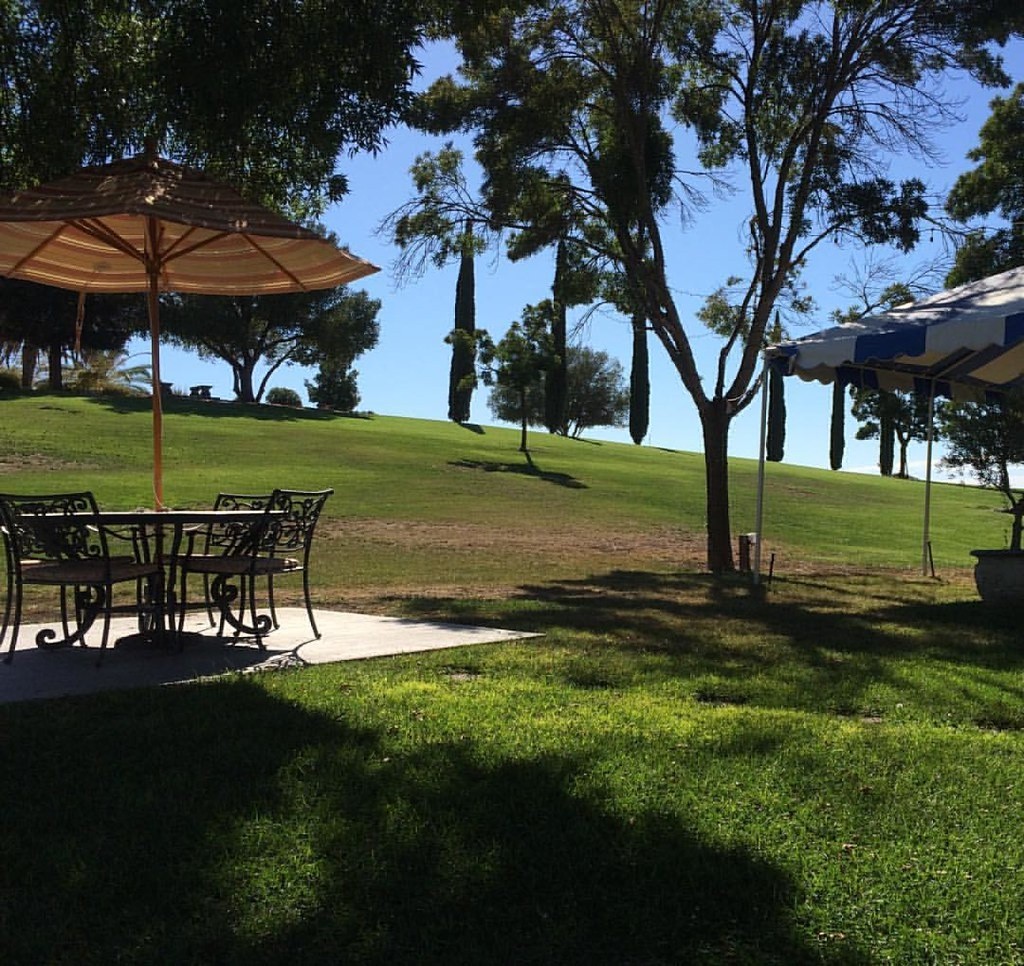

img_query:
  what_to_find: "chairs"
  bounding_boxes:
[0,486,337,667]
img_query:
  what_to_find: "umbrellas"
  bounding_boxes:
[0,147,380,511]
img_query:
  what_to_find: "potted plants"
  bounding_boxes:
[937,383,1024,623]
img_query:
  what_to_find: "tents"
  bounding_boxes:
[755,265,1024,595]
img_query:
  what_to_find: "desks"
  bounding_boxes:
[190,384,212,397]
[17,509,287,654]
[152,382,174,395]
